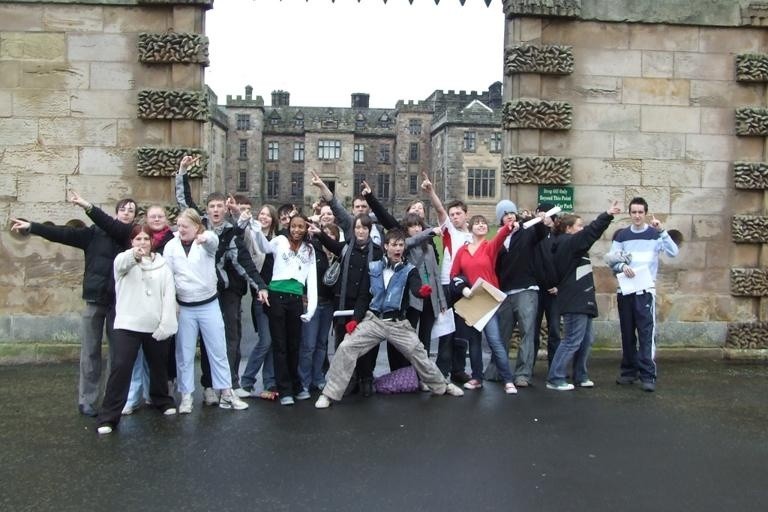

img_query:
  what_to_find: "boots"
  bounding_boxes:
[343,376,358,397]
[360,375,372,398]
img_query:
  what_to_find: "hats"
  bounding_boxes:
[536,203,556,221]
[495,199,517,224]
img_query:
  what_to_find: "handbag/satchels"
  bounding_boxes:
[322,260,342,287]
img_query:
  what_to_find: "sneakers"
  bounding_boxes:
[280,383,334,409]
[205,386,276,411]
[419,371,483,397]
[616,371,656,392]
[485,368,530,395]
[81,398,176,433]
[544,370,595,391]
[177,396,194,414]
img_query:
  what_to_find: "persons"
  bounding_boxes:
[605,196,679,392]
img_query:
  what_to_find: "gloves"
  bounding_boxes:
[419,284,433,298]
[345,320,358,335]
[462,286,472,298]
